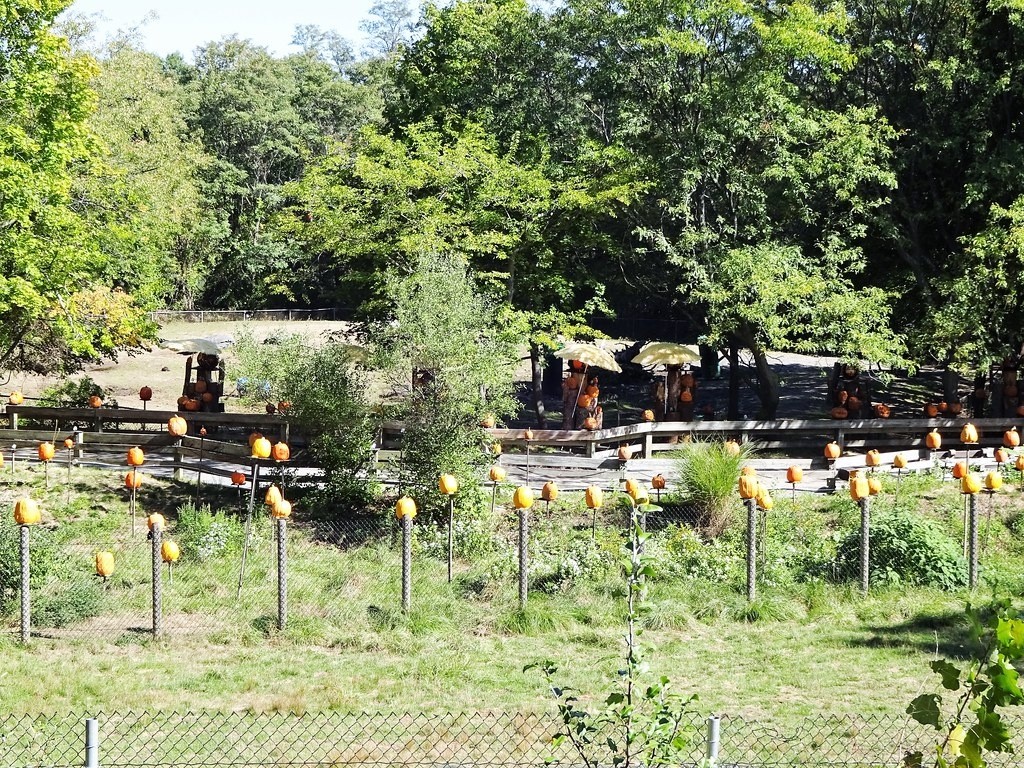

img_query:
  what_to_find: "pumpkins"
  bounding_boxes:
[7,359,1024,576]
